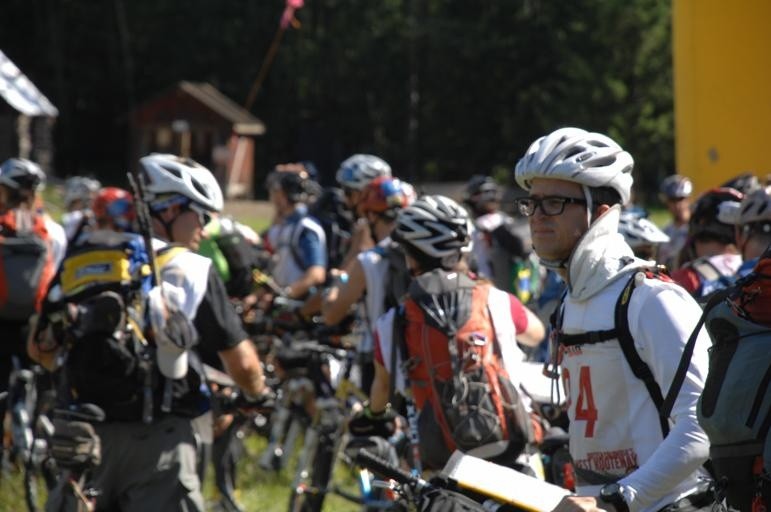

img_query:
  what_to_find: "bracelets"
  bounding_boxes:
[293,306,305,322]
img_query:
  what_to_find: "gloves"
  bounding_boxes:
[231,389,278,420]
[349,411,399,441]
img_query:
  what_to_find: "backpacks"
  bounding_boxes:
[399,278,541,473]
[33,237,210,429]
[475,211,544,304]
[214,219,255,299]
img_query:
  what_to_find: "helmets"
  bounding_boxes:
[688,188,744,239]
[390,193,477,258]
[2,155,48,200]
[130,145,225,219]
[263,166,310,207]
[734,188,770,233]
[513,126,635,209]
[335,156,393,194]
[659,175,692,202]
[58,177,97,210]
[356,176,418,220]
[92,186,136,229]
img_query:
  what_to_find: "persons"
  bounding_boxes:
[318,176,418,329]
[654,173,695,276]
[291,153,393,322]
[460,175,516,283]
[243,163,328,304]
[695,184,771,512]
[0,155,68,326]
[26,150,265,512]
[369,193,547,512]
[514,126,729,512]
[670,186,745,311]
[193,211,264,299]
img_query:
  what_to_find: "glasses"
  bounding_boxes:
[513,193,602,220]
[191,202,212,228]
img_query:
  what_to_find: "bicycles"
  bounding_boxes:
[0,269,490,511]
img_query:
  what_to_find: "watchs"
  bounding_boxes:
[599,481,632,512]
